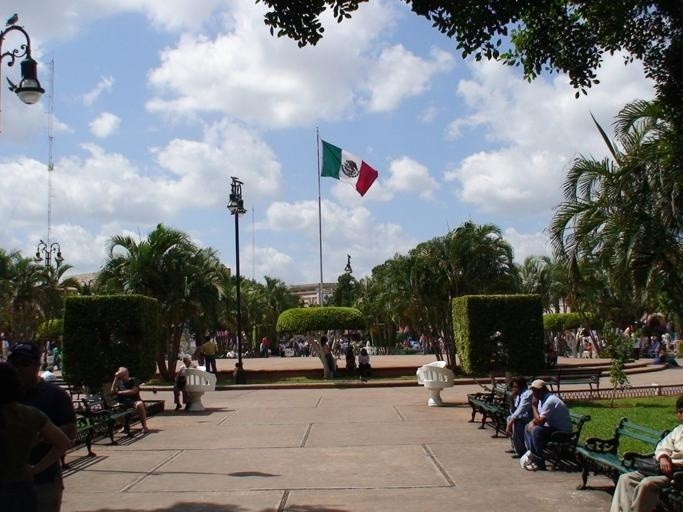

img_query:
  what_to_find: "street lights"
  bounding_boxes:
[0,12,45,105]
[227,176,247,384]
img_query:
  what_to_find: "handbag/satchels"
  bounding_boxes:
[519,451,534,468]
[634,455,676,477]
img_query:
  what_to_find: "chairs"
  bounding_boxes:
[422,364,454,408]
[181,366,206,414]
[415,360,447,407]
[183,369,215,413]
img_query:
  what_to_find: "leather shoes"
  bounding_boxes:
[511,453,523,458]
[505,448,516,452]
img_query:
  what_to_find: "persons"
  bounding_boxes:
[347,349,357,377]
[340,340,353,355]
[321,336,338,378]
[359,349,371,381]
[650,337,666,363]
[633,334,641,360]
[7,343,77,512]
[111,367,151,433]
[524,379,572,471]
[52,337,62,354]
[583,336,593,358]
[41,365,57,381]
[294,339,318,357]
[195,342,205,366]
[506,376,534,458]
[1,361,71,511]
[611,397,682,511]
[51,343,62,370]
[201,336,219,372]
[577,336,580,352]
[260,340,265,358]
[263,336,269,358]
[174,354,198,411]
[418,333,444,350]
[279,341,285,357]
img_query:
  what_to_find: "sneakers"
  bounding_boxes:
[525,463,546,471]
[185,402,190,410]
[174,404,182,412]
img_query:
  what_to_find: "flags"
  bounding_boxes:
[321,140,379,198]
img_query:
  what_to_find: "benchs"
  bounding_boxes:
[535,404,590,474]
[79,391,147,444]
[467,383,522,440]
[574,416,682,512]
[58,404,116,464]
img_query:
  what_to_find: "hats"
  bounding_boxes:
[529,379,546,389]
[8,342,41,364]
